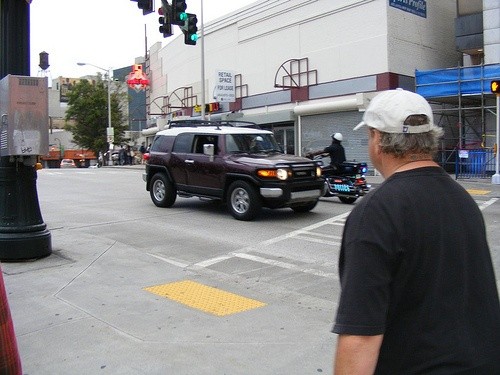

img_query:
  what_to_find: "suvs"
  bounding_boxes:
[142,120,328,222]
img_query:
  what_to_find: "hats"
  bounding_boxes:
[354,87,434,134]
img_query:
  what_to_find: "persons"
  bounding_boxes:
[98,156,102,168]
[120,142,152,165]
[330,88,500,375]
[0,267,23,375]
[205,135,252,153]
[304,133,346,196]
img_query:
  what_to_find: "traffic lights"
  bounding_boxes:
[158,5,172,38]
[172,0,188,26]
[129,0,155,15]
[184,13,198,45]
[491,80,500,93]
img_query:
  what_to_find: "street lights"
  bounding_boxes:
[77,62,114,166]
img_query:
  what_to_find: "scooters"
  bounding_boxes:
[302,147,372,204]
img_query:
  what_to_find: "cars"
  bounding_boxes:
[87,163,99,168]
[60,159,80,168]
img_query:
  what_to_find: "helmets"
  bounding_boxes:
[330,132,343,141]
